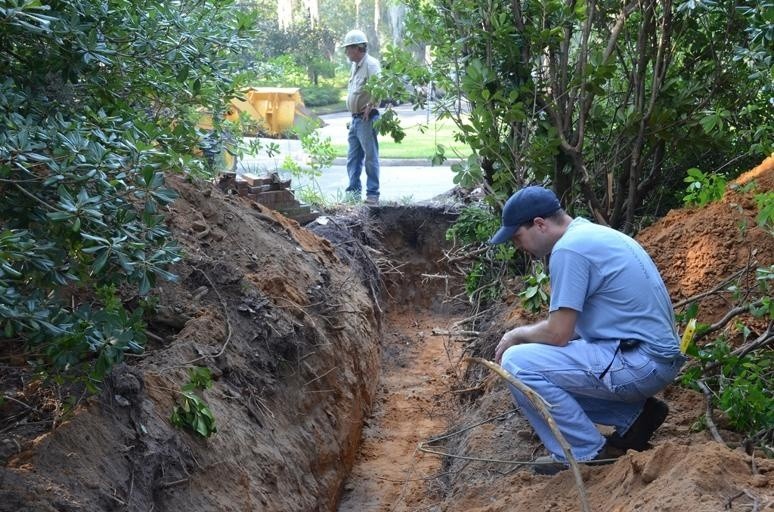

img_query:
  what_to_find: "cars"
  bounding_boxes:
[379,72,418,108]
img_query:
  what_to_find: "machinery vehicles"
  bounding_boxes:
[192,86,329,173]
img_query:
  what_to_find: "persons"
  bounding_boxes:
[340,29,382,205]
[489,186,686,475]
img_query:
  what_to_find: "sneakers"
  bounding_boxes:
[610,397,669,451]
[364,196,379,204]
[535,449,614,476]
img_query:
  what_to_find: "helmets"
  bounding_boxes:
[340,29,367,49]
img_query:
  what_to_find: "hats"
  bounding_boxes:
[492,186,559,244]
